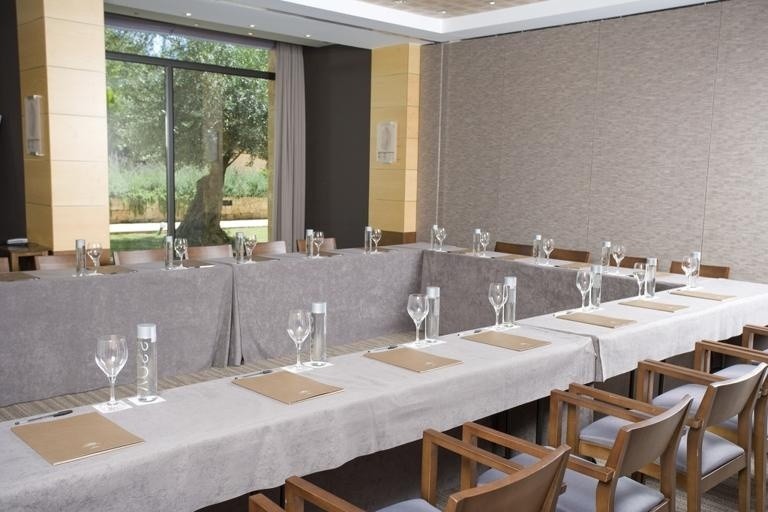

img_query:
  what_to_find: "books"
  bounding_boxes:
[237,253,280,263]
[10,410,145,470]
[362,244,399,254]
[233,368,344,409]
[492,252,531,262]
[560,260,592,270]
[0,270,40,283]
[449,246,479,256]
[556,311,636,331]
[669,289,736,303]
[173,258,216,270]
[617,298,689,315]
[301,249,344,258]
[462,329,551,354]
[655,271,672,282]
[87,264,137,275]
[364,346,462,377]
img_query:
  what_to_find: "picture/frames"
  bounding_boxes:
[376,121,398,165]
[24,93,43,157]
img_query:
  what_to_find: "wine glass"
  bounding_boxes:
[174,238,188,270]
[430,223,628,271]
[94,333,131,409]
[372,229,383,255]
[84,240,104,274]
[284,309,313,374]
[633,262,647,301]
[680,256,697,295]
[575,268,594,314]
[488,282,509,331]
[405,293,430,349]
[314,232,325,258]
[244,233,257,263]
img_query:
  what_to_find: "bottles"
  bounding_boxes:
[309,300,328,368]
[503,275,518,329]
[305,229,314,258]
[424,286,441,343]
[74,239,87,276]
[164,235,174,269]
[689,251,701,292]
[365,227,372,254]
[234,231,245,264]
[589,265,603,310]
[644,258,657,300]
[136,321,158,405]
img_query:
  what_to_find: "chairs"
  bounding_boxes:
[646,341,768,512]
[187,244,234,259]
[702,327,768,379]
[545,248,590,263]
[248,493,282,512]
[84,248,119,267]
[566,360,768,512]
[2,257,9,272]
[609,254,647,268]
[295,239,337,252]
[284,428,572,512]
[746,323,768,353]
[670,260,731,278]
[253,241,287,256]
[460,389,695,512]
[494,242,538,257]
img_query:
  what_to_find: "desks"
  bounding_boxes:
[419,247,709,335]
[3,241,50,271]
[515,277,768,428]
[204,241,466,366]
[1,322,596,512]
[3,258,233,408]
